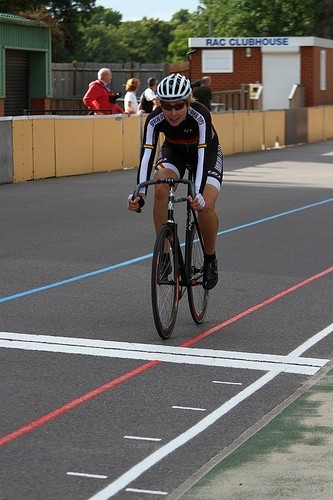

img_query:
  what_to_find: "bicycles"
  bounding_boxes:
[132,163,211,341]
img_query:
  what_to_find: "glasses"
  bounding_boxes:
[161,103,185,111]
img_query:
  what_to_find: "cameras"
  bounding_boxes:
[108,92,121,104]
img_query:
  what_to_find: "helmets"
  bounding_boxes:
[157,73,191,101]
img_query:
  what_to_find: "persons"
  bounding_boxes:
[82,68,131,117]
[128,72,223,289]
[138,77,163,115]
[191,75,214,112]
[124,78,145,116]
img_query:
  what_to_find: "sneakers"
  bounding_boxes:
[204,257,218,290]
[158,263,172,284]
